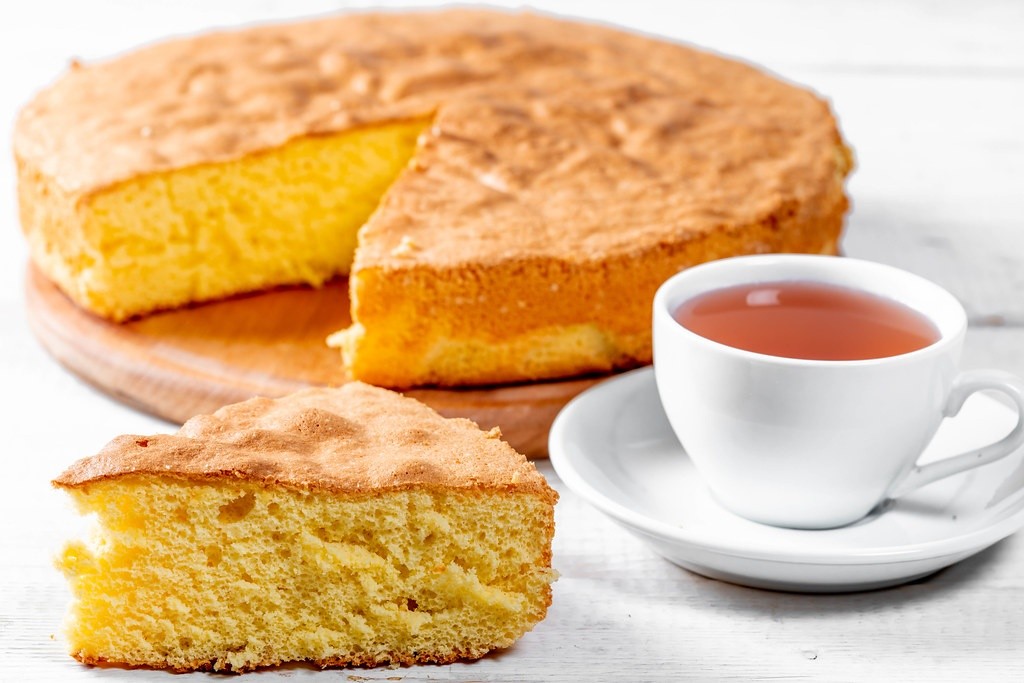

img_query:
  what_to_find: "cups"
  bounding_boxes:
[652,252,1024,529]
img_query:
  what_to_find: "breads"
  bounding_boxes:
[54,382,557,673]
[15,8,852,388]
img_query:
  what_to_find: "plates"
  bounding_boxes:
[546,366,1024,594]
[19,257,616,462]
